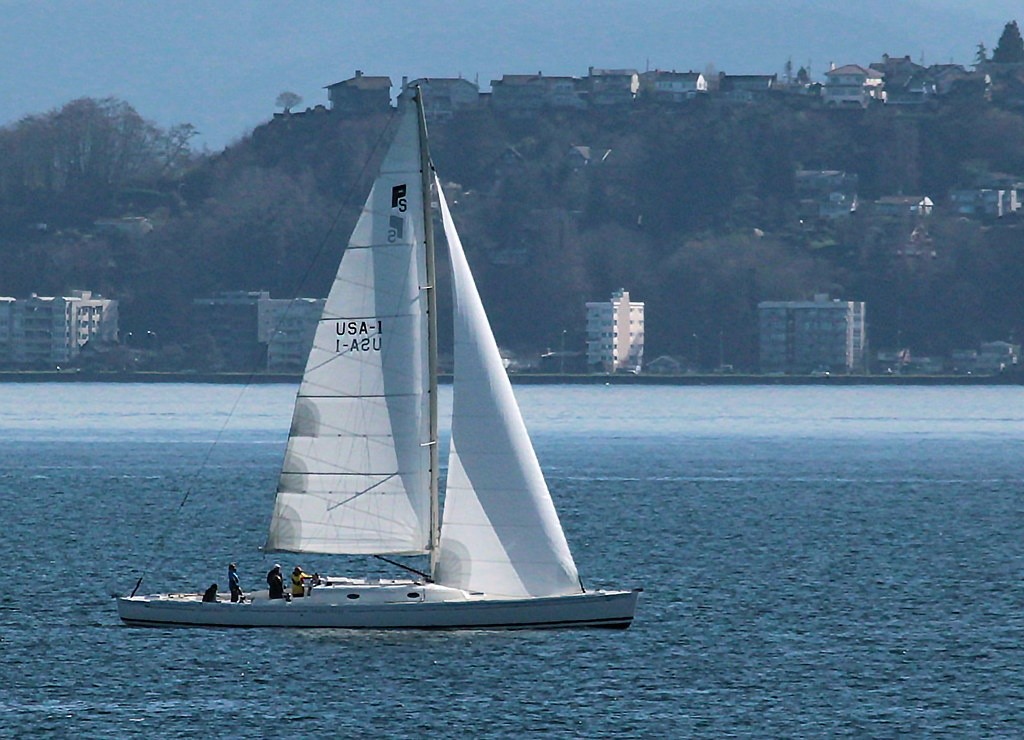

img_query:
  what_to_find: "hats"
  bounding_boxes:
[294,566,305,572]
[274,564,281,568]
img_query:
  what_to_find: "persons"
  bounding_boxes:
[202,584,218,602]
[267,564,283,599]
[228,563,242,602]
[291,567,313,597]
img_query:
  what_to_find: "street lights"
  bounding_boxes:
[561,329,567,373]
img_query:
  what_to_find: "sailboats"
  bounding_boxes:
[109,108,643,633]
